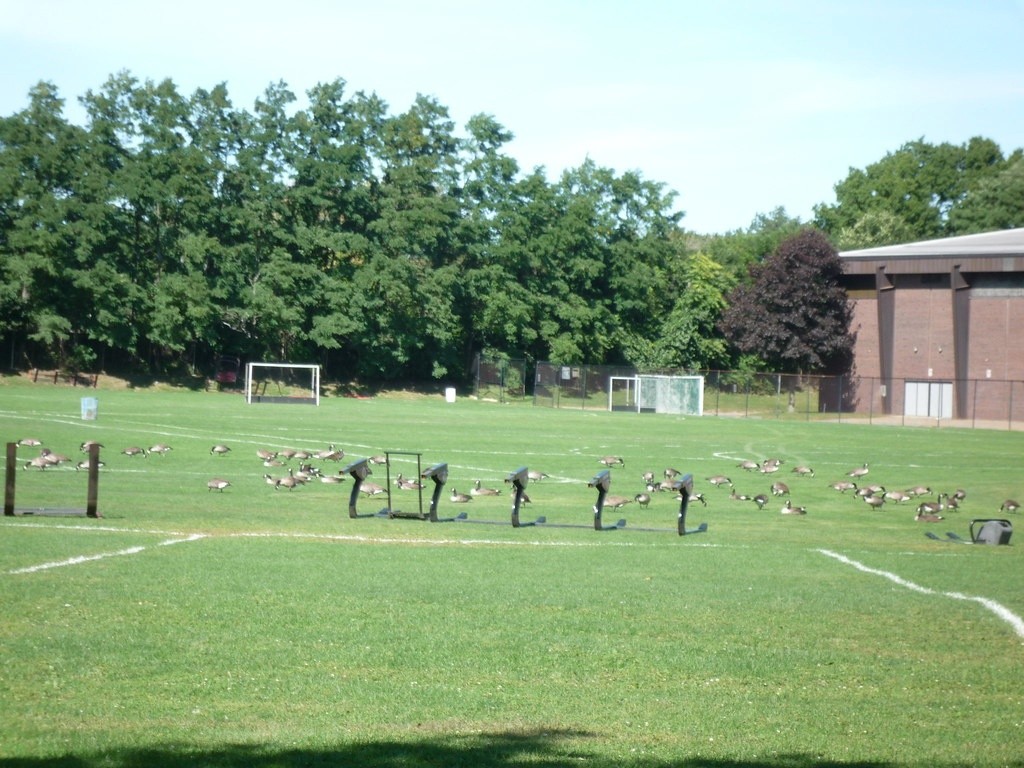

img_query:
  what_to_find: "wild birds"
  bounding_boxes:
[147,445,172,457]
[449,488,473,502]
[509,484,532,507]
[597,456,625,468]
[76,460,106,471]
[256,444,346,491]
[396,474,426,490]
[368,456,387,466]
[359,482,388,498]
[642,458,1021,524]
[16,440,43,448]
[635,493,650,509]
[80,441,106,454]
[121,447,147,459]
[210,445,232,457]
[528,471,549,484]
[471,480,502,497]
[23,447,72,471]
[207,478,231,493]
[593,496,632,513]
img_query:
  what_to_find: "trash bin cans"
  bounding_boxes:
[80,397,98,420]
[446,388,456,403]
